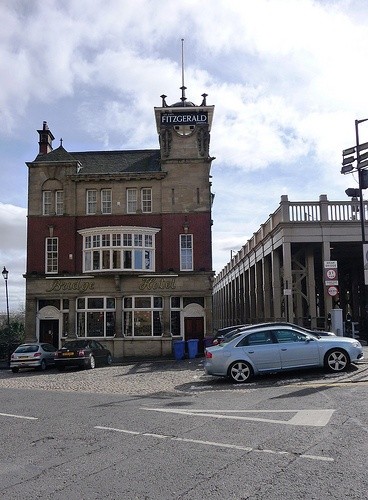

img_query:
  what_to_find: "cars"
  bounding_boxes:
[53,340,113,370]
[10,342,58,372]
[203,321,363,385]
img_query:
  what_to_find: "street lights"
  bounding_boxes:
[1,266,12,368]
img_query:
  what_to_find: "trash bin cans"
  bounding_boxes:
[173,340,185,361]
[187,339,200,359]
[202,337,213,357]
